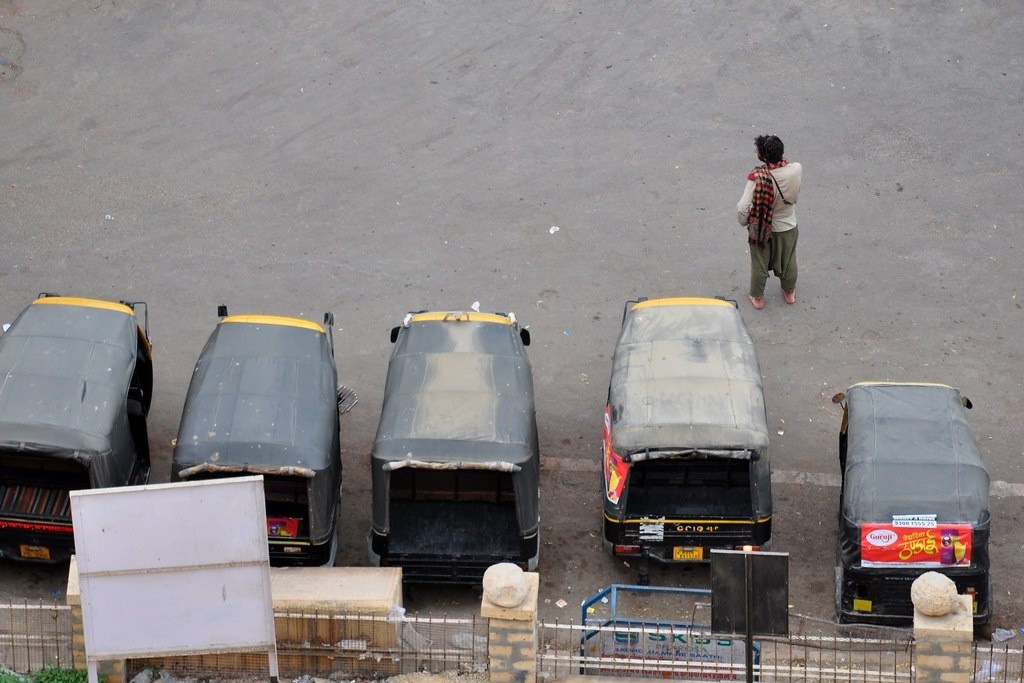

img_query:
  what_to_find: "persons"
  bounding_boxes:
[737,133,803,310]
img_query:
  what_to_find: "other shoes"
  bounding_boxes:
[749,294,764,309]
[784,290,796,304]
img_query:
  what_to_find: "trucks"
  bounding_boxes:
[0,292,152,575]
[171,304,343,571]
[371,310,544,592]
[829,380,996,641]
[603,296,773,576]
